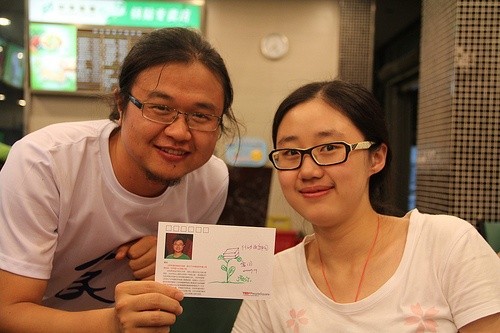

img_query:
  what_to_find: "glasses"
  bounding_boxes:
[269,140,377,171]
[122,89,222,131]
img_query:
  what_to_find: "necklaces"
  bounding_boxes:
[316,215,384,305]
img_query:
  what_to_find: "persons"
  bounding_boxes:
[230,79,500,333]
[165,236,191,260]
[0,26,230,333]
